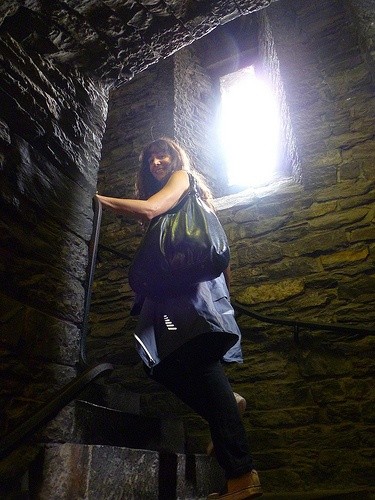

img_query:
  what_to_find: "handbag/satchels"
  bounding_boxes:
[127,173,230,294]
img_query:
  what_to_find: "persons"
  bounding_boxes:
[96,139,263,500]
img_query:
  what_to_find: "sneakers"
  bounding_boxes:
[206,392,247,456]
[207,468,263,500]
